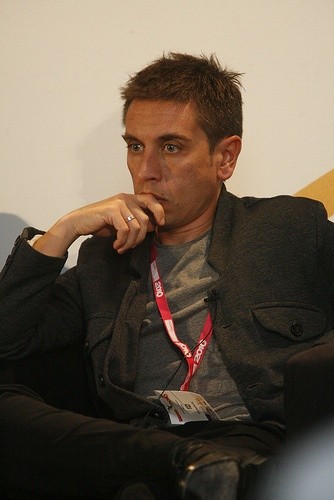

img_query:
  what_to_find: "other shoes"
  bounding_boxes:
[177,440,272,500]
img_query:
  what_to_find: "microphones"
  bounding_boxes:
[212,289,217,296]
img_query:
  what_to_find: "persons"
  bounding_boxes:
[0,53,334,500]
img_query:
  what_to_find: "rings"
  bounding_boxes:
[124,215,134,223]
